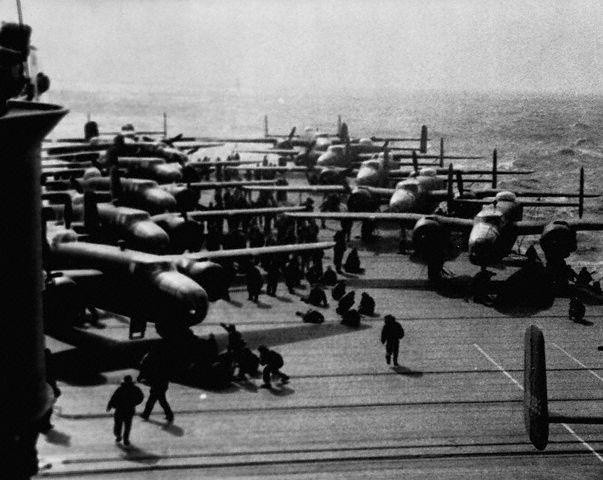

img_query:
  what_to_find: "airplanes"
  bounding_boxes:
[43,112,603,338]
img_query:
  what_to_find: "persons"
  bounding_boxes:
[135,347,174,425]
[564,265,601,323]
[525,243,538,261]
[105,372,144,448]
[470,266,497,295]
[380,314,405,366]
[333,204,363,275]
[208,322,290,390]
[197,153,325,300]
[296,266,376,329]
[319,197,331,229]
